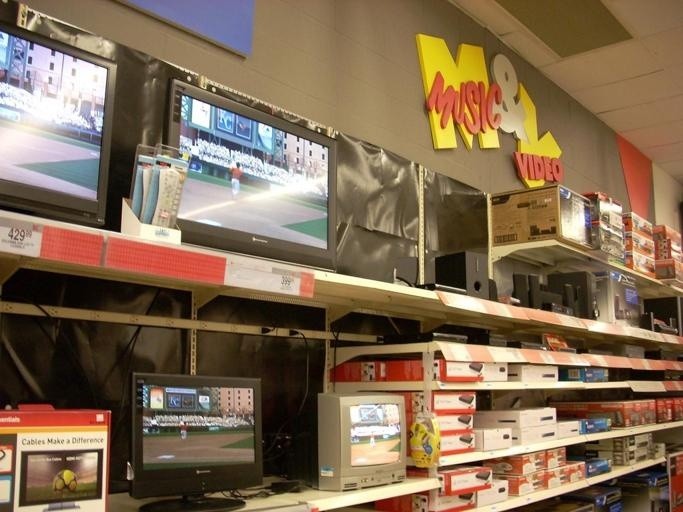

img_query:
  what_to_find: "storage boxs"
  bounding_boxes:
[492,183,593,252]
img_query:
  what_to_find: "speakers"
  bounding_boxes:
[641,296,683,336]
[435,251,489,300]
[489,269,600,320]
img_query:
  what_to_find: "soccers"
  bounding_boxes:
[52,470,77,492]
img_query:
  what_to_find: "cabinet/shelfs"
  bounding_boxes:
[0,209,683,512]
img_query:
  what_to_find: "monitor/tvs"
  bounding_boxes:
[292,392,407,491]
[20,449,103,511]
[0,19,118,228]
[129,371,263,512]
[163,77,338,274]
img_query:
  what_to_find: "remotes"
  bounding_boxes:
[130,165,188,228]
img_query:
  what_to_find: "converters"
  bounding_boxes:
[271,481,299,492]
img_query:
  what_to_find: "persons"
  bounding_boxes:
[229,160,246,201]
[180,422,188,440]
[369,430,375,447]
[188,143,200,171]
